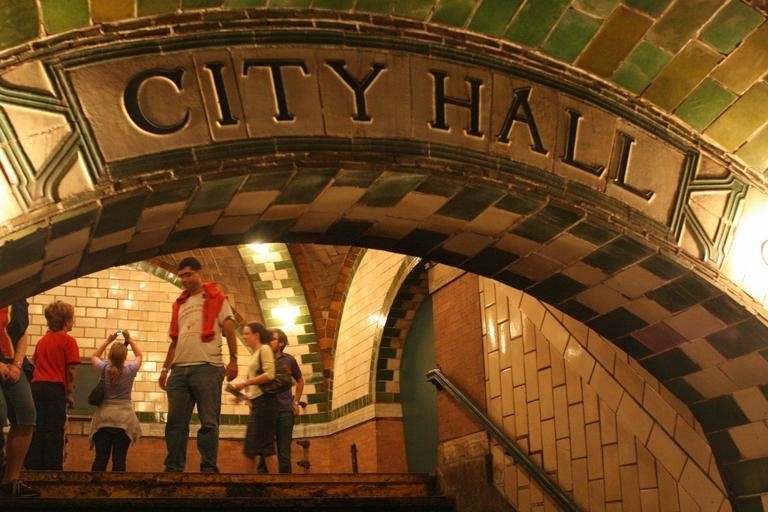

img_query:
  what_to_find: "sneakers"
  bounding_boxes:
[0,479,41,496]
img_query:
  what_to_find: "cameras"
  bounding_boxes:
[116,333,123,336]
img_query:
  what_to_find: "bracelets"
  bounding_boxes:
[232,354,237,359]
[161,368,168,372]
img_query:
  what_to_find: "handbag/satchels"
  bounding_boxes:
[88,365,107,406]
[256,344,292,396]
[23,356,34,381]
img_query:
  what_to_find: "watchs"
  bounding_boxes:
[13,361,21,369]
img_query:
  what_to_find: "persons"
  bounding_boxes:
[158,257,238,473]
[29,300,81,471]
[89,328,142,470]
[0,298,41,498]
[235,321,304,474]
